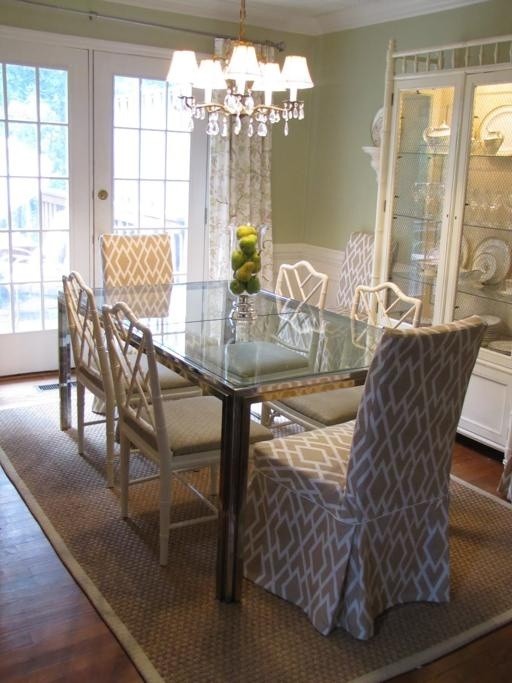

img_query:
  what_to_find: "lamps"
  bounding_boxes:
[166,0,315,138]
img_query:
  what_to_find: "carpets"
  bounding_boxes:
[0,383,512,683]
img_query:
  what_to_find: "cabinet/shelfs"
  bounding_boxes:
[365,35,512,468]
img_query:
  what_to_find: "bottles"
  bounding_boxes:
[484,129,504,156]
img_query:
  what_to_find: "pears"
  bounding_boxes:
[230,225,262,294]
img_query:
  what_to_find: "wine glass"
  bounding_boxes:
[410,181,512,231]
[228,221,267,321]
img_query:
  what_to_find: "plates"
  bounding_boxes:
[471,313,512,356]
[479,106,512,153]
[470,235,511,285]
